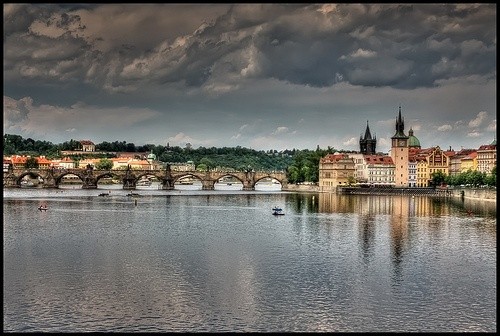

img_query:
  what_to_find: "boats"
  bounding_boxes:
[38,206,48,210]
[272,206,285,216]
